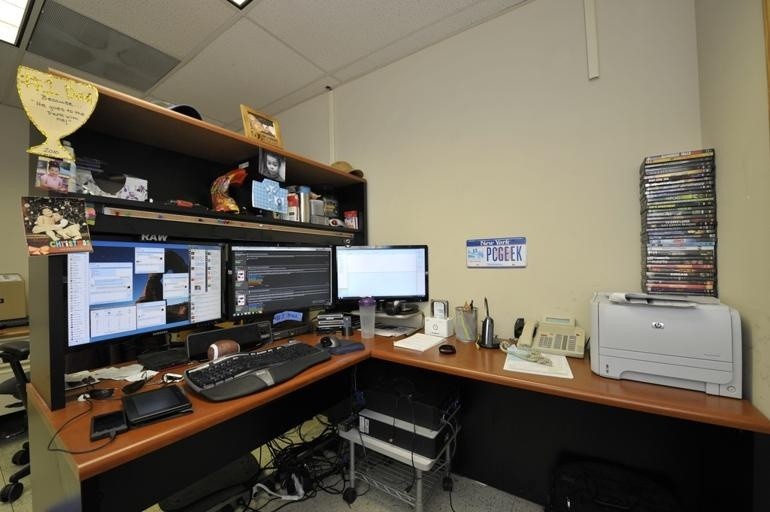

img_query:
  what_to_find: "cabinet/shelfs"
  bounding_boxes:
[24,65,422,512]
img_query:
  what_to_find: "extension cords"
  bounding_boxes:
[205,489,253,512]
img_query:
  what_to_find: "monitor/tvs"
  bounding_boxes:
[337,245,435,311]
[227,244,338,327]
[59,240,223,371]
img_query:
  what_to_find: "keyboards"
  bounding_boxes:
[186,339,331,398]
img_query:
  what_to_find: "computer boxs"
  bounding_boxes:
[359,379,464,458]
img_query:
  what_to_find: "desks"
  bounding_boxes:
[370,324,770,512]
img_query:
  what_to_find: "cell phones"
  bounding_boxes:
[90,411,127,440]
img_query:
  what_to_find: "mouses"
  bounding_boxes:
[321,336,341,349]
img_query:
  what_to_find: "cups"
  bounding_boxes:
[455,306,479,343]
[359,299,375,339]
[297,185,311,224]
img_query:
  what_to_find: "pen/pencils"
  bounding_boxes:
[459,301,477,340]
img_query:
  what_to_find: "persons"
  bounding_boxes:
[32,206,82,242]
[263,152,284,182]
[251,120,276,136]
[39,161,64,190]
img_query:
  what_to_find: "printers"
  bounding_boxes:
[590,293,742,400]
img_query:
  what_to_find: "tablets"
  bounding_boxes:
[122,383,192,424]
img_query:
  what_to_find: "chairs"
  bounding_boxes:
[0,340,33,503]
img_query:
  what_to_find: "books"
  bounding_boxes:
[395,330,444,353]
[639,147,718,298]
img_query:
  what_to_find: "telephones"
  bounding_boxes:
[517,314,585,359]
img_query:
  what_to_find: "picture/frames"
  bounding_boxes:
[240,103,284,149]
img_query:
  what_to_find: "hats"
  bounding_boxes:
[332,162,363,177]
